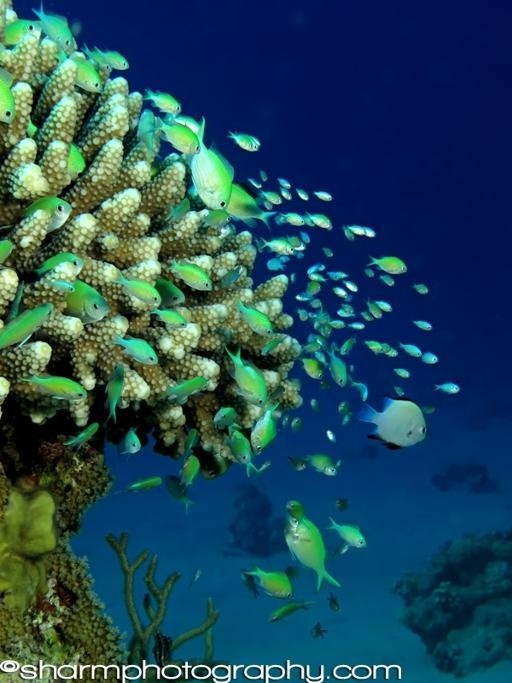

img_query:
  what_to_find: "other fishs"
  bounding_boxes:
[0,0,462,641]
